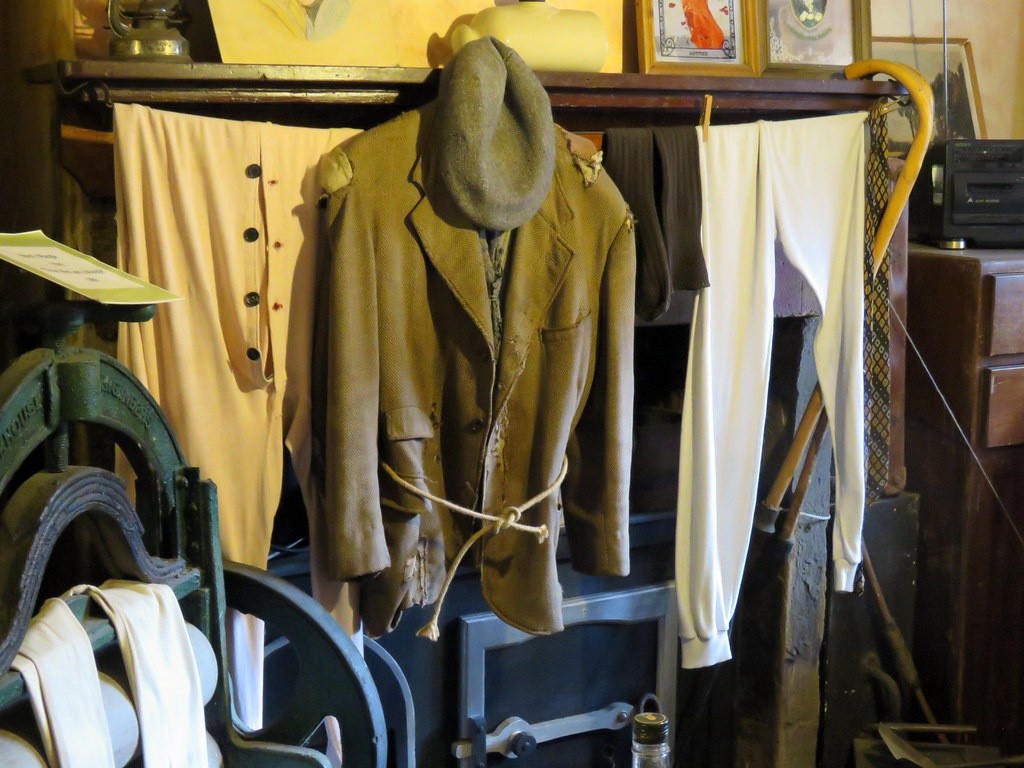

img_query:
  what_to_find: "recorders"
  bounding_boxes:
[910,138,1024,248]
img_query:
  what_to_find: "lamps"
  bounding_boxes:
[105,0,194,65]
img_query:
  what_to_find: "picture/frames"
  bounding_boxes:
[634,0,873,80]
[870,35,987,158]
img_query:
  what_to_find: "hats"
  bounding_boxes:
[435,35,556,232]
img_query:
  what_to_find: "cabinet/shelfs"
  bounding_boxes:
[906,242,1024,751]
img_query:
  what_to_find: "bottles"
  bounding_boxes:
[630,712,672,768]
